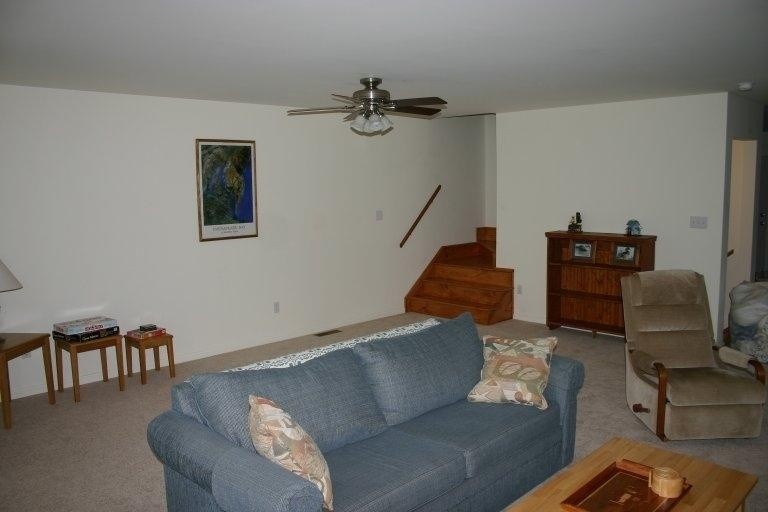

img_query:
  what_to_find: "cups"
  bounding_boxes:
[648,467,682,499]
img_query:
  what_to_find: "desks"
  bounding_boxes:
[0,332,56,429]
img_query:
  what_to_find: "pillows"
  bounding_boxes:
[187,347,388,454]
[355,310,484,428]
[247,392,335,512]
[465,334,559,409]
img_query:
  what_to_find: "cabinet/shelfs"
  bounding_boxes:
[545,230,658,340]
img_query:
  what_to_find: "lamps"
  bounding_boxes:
[0,259,23,343]
[350,77,395,136]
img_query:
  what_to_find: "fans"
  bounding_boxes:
[286,93,448,119]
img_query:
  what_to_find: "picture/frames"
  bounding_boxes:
[195,139,258,242]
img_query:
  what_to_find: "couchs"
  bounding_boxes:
[147,310,584,512]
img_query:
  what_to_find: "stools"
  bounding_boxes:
[124,334,176,385]
[55,335,126,402]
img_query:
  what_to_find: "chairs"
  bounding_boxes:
[721,281,766,392]
[620,269,767,442]
